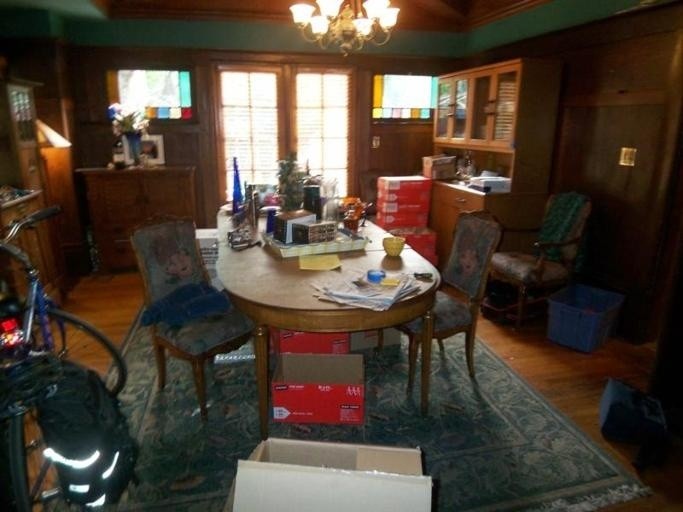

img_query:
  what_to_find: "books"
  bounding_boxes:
[493,79,519,141]
[259,225,371,258]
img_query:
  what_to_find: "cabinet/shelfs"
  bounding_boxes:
[1,193,62,306]
[471,60,565,149]
[431,175,549,261]
[6,79,64,284]
[79,164,199,270]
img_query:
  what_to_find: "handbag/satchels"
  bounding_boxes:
[600,377,667,444]
[36,360,137,507]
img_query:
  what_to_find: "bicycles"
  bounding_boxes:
[0,204,126,512]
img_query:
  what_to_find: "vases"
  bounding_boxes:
[124,134,143,169]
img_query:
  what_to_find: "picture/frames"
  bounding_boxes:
[121,135,170,165]
[433,70,470,146]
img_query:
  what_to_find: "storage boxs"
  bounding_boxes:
[268,351,366,424]
[221,437,437,512]
[375,173,439,266]
[269,327,351,354]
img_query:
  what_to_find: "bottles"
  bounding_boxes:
[485,153,495,173]
[111,136,125,170]
[343,210,358,231]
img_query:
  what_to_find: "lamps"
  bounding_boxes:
[616,146,635,209]
[367,132,380,155]
[284,0,403,58]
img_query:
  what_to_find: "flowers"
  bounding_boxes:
[107,102,150,142]
[275,157,306,211]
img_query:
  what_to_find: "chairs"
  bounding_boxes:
[482,194,595,325]
[131,211,254,424]
[376,208,502,405]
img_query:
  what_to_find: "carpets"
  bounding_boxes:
[40,300,648,511]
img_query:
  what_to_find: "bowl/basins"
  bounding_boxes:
[378,254,404,271]
[381,236,406,256]
